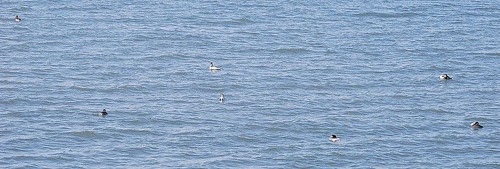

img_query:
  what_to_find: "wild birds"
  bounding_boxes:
[440,74,452,80]
[15,15,21,21]
[219,93,224,102]
[99,108,108,115]
[471,121,484,128]
[209,61,220,70]
[329,134,339,142]
